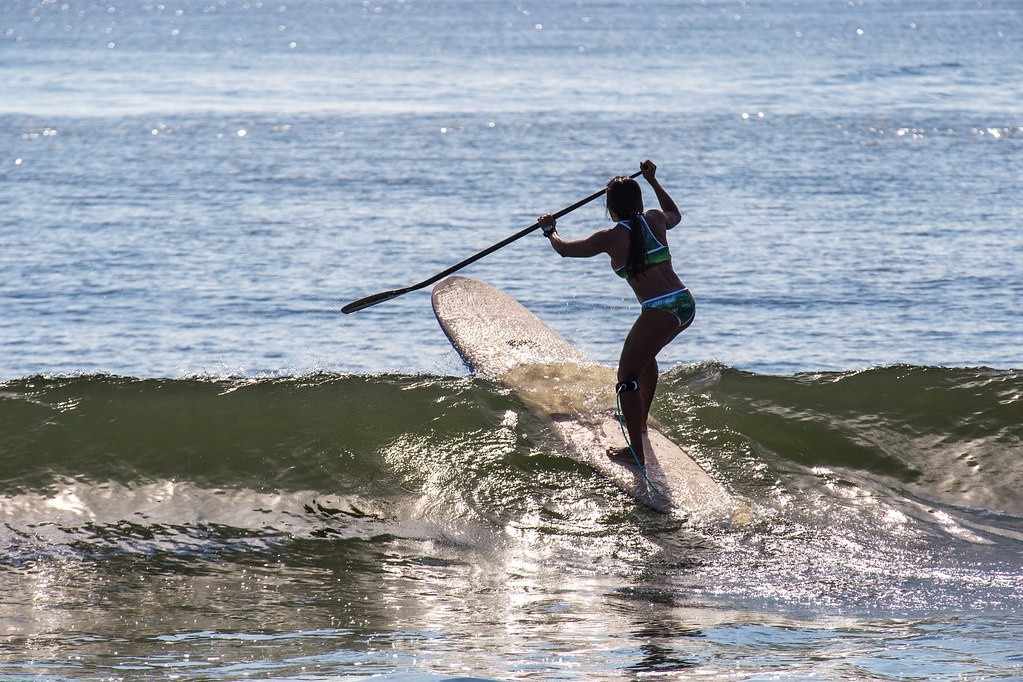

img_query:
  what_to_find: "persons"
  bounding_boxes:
[537,159,696,465]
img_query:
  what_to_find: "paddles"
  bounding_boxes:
[340,165,649,315]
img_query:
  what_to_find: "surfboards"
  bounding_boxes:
[431,275,756,526]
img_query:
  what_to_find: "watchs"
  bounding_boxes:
[541,225,556,236]
